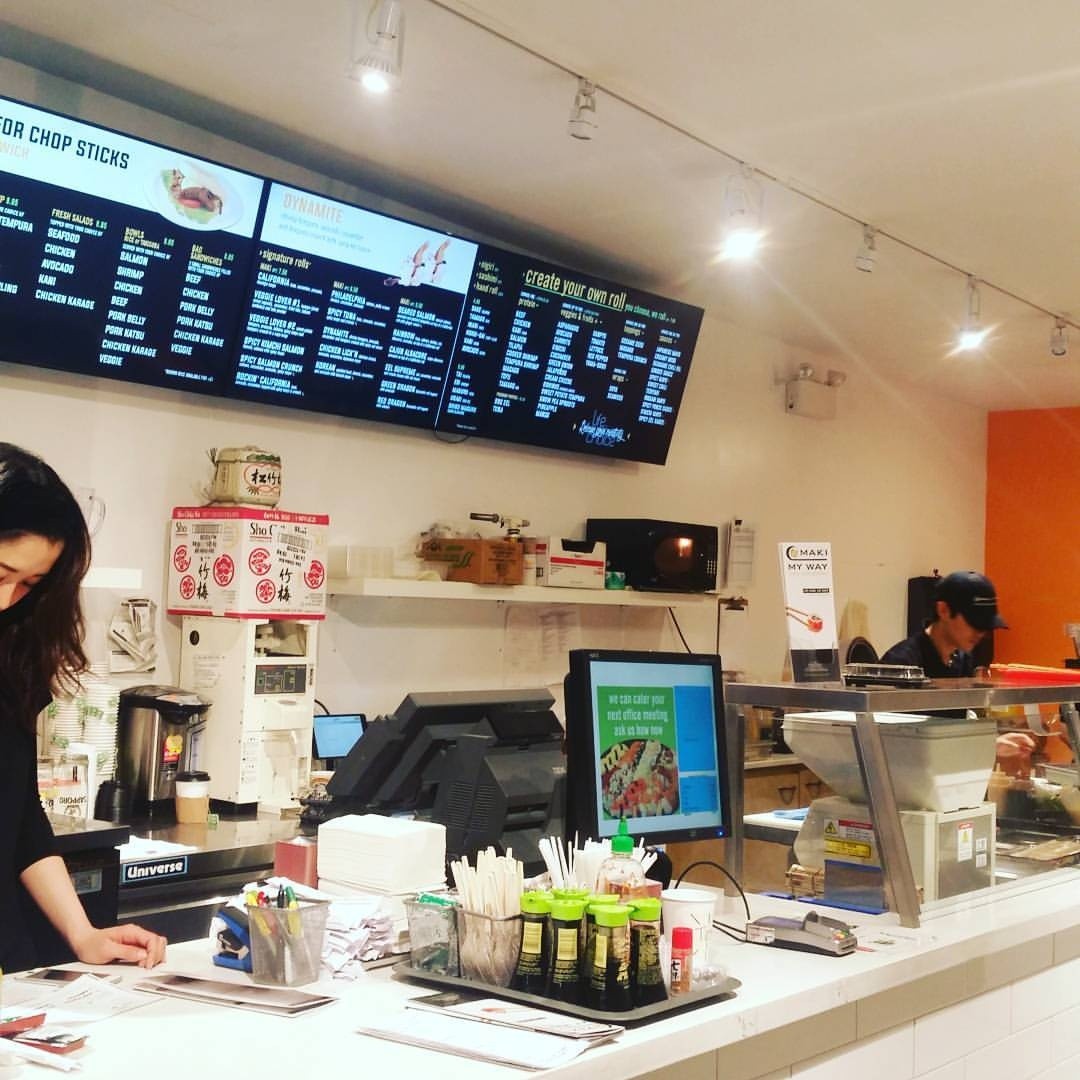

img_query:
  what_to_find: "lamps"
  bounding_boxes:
[343,0,410,92]
[785,362,846,420]
[960,274,984,337]
[1048,318,1070,359]
[567,78,599,141]
[725,160,759,248]
[854,224,880,274]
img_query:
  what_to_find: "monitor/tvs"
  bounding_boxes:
[223,177,481,432]
[1,94,270,398]
[433,242,705,466]
[563,648,732,892]
[312,713,367,770]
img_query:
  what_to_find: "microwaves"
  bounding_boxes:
[586,518,718,592]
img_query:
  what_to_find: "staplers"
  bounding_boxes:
[214,905,252,972]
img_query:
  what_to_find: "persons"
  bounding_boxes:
[878,570,1035,757]
[0,442,169,970]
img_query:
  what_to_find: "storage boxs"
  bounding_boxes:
[423,536,522,586]
[535,536,608,590]
[163,505,332,619]
[329,543,396,578]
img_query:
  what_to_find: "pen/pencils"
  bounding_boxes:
[245,881,315,984]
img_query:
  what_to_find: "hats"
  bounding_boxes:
[932,569,1009,631]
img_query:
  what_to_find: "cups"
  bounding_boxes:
[661,889,716,975]
[175,772,210,823]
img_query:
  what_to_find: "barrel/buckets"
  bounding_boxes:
[117,685,212,818]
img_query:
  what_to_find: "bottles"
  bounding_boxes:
[987,761,1036,818]
[511,816,694,1012]
